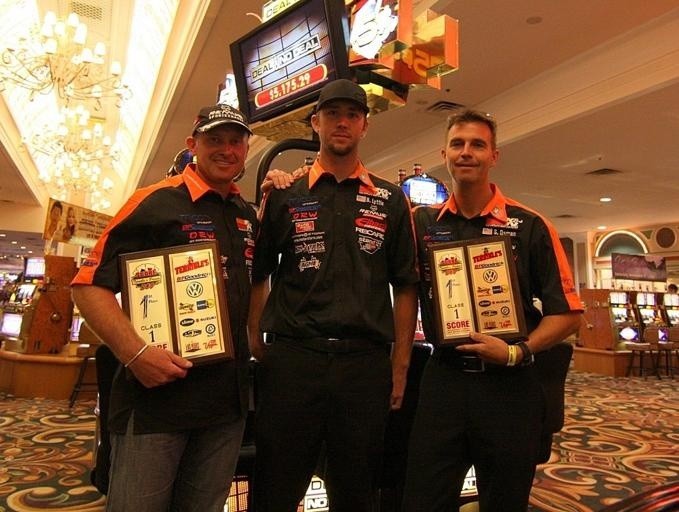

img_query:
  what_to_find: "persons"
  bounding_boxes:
[664,284,679,305]
[63,207,77,240]
[47,202,62,236]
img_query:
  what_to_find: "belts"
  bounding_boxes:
[310,332,378,354]
[438,350,507,378]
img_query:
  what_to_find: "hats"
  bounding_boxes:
[316,79,370,115]
[191,103,254,137]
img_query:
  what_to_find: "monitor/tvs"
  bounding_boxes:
[14,284,37,302]
[69,315,85,342]
[0,312,23,338]
[610,291,679,344]
[228,0,356,125]
[24,258,45,278]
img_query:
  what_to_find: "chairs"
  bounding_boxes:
[68,321,104,409]
[624,326,679,382]
